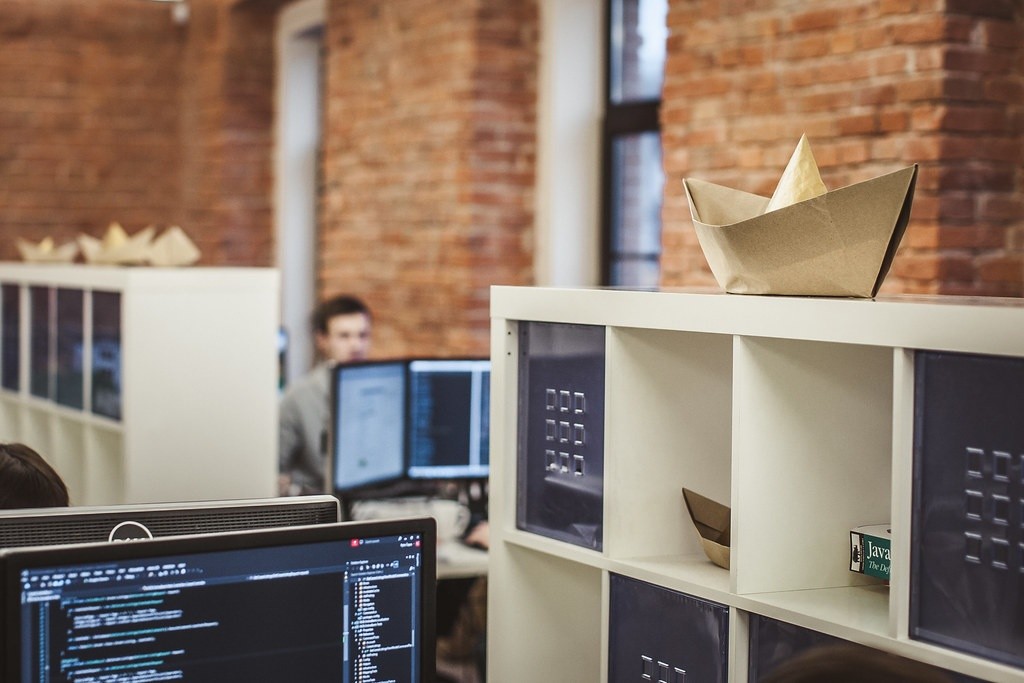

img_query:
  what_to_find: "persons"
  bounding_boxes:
[277,293,487,661]
[1,444,71,512]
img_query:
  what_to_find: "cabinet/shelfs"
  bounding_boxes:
[486,285,1024,683]
[0,260,281,511]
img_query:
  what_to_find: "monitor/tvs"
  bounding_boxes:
[0,351,491,683]
